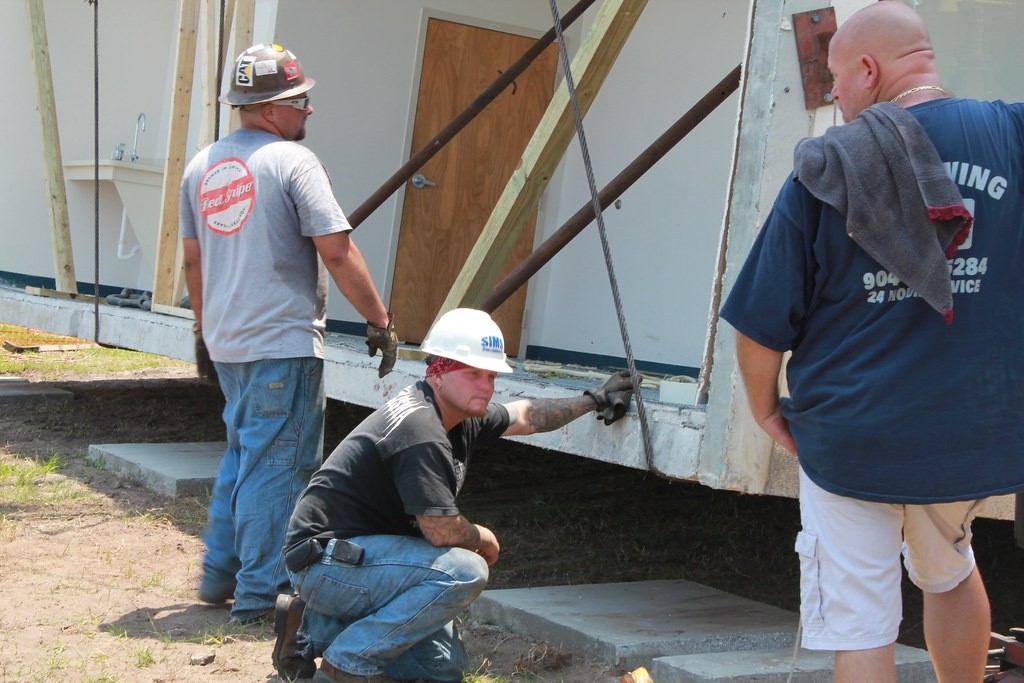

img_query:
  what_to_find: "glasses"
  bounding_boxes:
[270,96,310,110]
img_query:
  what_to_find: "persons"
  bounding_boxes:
[271,309,642,683]
[177,42,399,627]
[723,0,1024,682]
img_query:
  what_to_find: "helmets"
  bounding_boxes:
[218,43,316,105]
[422,308,513,374]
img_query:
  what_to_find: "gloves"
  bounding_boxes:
[193,322,219,384]
[365,312,398,378]
[584,370,643,426]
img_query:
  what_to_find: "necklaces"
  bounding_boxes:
[890,84,947,104]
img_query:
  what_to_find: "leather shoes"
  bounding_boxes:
[271,594,317,681]
[313,657,396,683]
